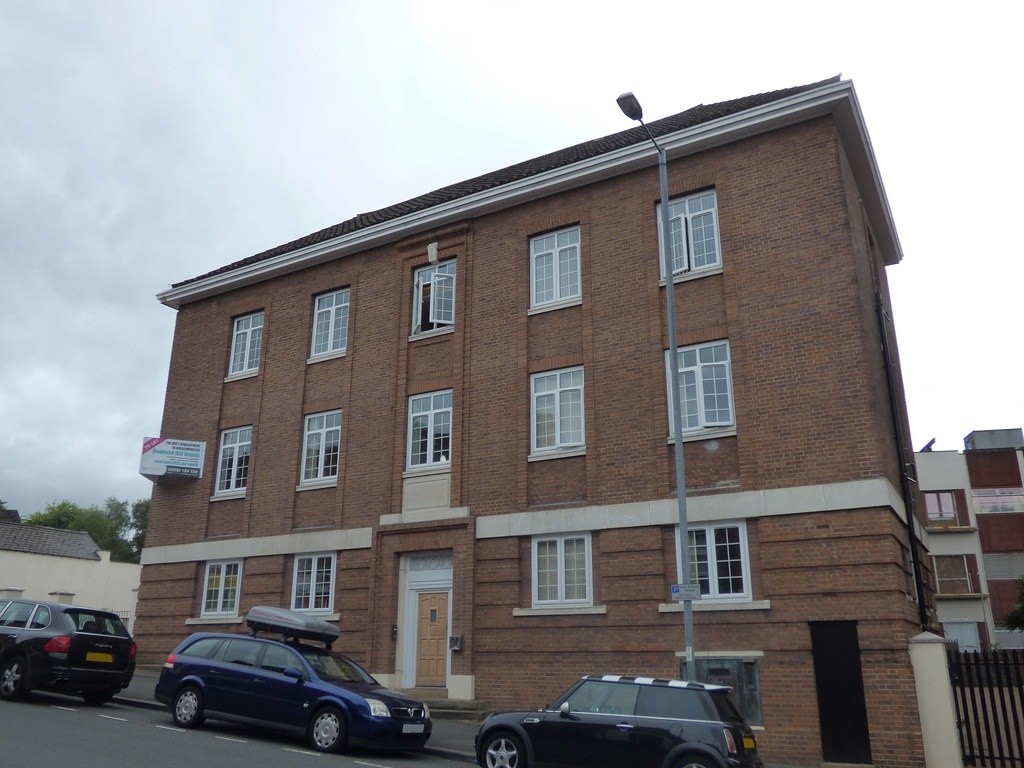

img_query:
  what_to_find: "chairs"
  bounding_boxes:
[82,621,99,634]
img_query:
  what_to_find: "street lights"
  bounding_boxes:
[615,92,698,685]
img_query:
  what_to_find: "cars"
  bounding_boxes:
[153,604,435,753]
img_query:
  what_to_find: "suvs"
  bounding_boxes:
[475,673,761,768]
[0,597,137,707]
